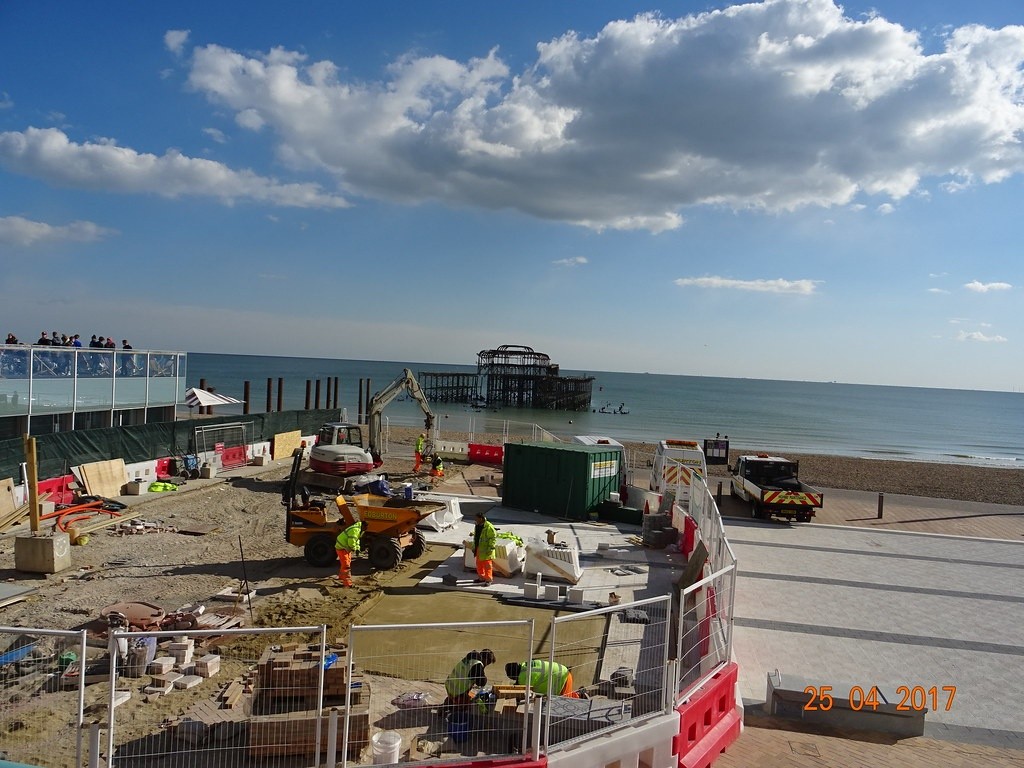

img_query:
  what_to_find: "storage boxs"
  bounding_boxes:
[500,441,624,522]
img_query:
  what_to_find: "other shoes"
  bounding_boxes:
[474,578,484,582]
[484,582,491,586]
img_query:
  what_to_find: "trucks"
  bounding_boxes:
[727,453,824,523]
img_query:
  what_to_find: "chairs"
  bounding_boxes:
[2,353,176,378]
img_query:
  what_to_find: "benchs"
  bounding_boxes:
[766,668,930,737]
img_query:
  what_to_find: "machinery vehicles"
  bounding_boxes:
[294,368,435,496]
[286,440,448,571]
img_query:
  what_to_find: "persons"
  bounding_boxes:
[37,332,83,376]
[445,648,496,708]
[89,333,132,375]
[335,521,370,588]
[472,512,496,587]
[505,659,587,699]
[430,452,444,476]
[6,333,17,370]
[412,433,426,472]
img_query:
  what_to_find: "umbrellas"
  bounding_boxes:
[185,387,246,417]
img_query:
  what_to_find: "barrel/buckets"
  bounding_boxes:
[446,712,469,743]
[371,731,401,766]
[67,527,79,545]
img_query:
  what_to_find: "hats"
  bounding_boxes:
[420,433,425,438]
[42,331,47,335]
[92,334,96,339]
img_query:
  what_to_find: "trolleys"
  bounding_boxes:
[166,446,190,480]
[178,445,202,481]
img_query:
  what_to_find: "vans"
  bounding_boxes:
[646,439,707,506]
